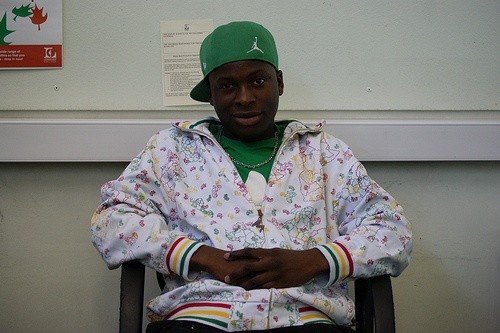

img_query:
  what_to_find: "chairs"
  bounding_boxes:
[117,246,396,333]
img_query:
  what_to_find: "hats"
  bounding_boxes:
[190,21,278,102]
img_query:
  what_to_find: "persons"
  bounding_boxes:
[89,20,412,333]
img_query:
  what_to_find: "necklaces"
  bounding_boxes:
[218,121,279,169]
